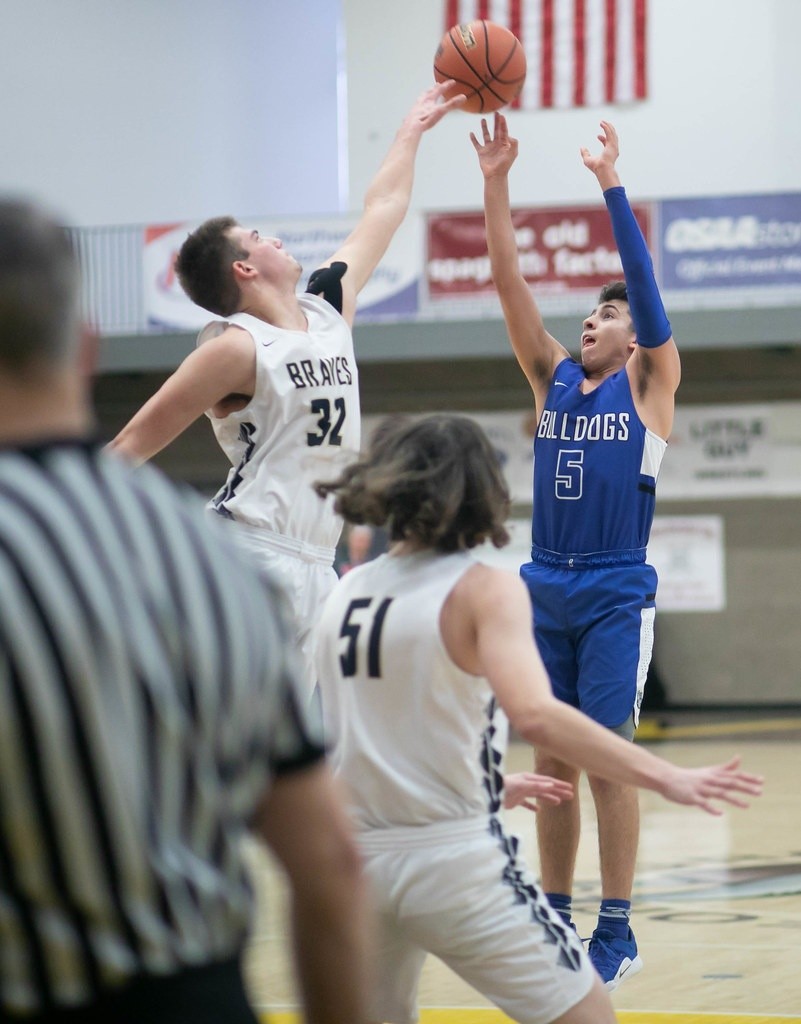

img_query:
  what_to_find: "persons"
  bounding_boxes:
[101,79,465,671]
[304,414,762,1023]
[464,111,685,984]
[0,187,373,1024]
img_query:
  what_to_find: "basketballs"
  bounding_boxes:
[432,17,529,117]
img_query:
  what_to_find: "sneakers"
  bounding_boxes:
[578,928,640,988]
